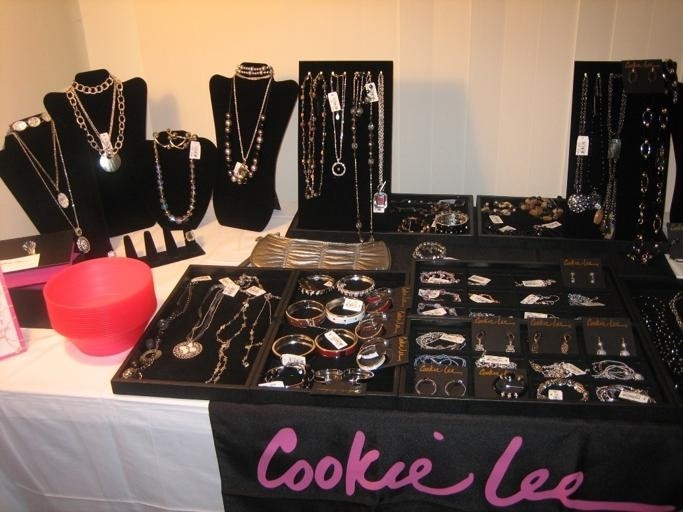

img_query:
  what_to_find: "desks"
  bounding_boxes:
[0,205,683,512]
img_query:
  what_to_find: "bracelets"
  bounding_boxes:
[391,194,571,234]
[414,241,657,411]
[266,271,391,395]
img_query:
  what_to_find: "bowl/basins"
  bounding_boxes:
[44,256,159,357]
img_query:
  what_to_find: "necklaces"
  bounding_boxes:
[13,122,92,256]
[301,72,385,234]
[67,73,126,176]
[121,271,281,390]
[13,122,70,208]
[148,129,196,224]
[567,71,632,241]
[225,66,273,185]
